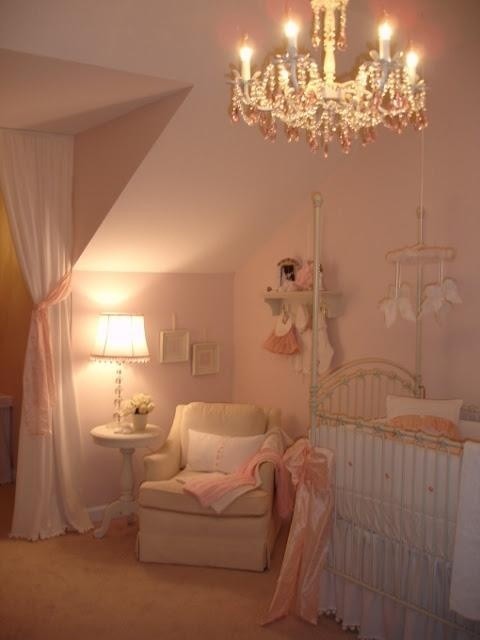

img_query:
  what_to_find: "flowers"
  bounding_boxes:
[122,391,157,420]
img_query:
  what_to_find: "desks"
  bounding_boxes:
[90,421,166,546]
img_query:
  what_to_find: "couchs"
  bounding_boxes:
[135,402,285,567]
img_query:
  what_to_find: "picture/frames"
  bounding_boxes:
[188,338,223,378]
[158,326,192,364]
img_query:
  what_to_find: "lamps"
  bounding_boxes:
[87,308,156,435]
[225,1,432,165]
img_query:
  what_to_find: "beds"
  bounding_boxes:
[307,191,480,640]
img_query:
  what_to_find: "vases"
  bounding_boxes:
[130,410,151,433]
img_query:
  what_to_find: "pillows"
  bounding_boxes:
[184,428,268,478]
